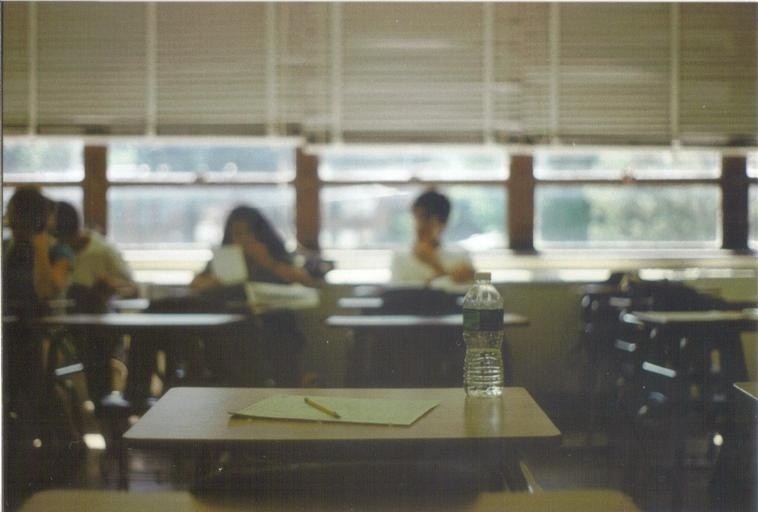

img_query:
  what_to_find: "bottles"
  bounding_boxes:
[461,272,506,399]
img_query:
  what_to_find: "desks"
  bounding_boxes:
[1,286,758,512]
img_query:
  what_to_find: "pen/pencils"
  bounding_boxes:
[304,397,341,418]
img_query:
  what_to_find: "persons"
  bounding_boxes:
[3,184,69,309]
[390,189,475,288]
[189,208,312,385]
[50,200,139,299]
